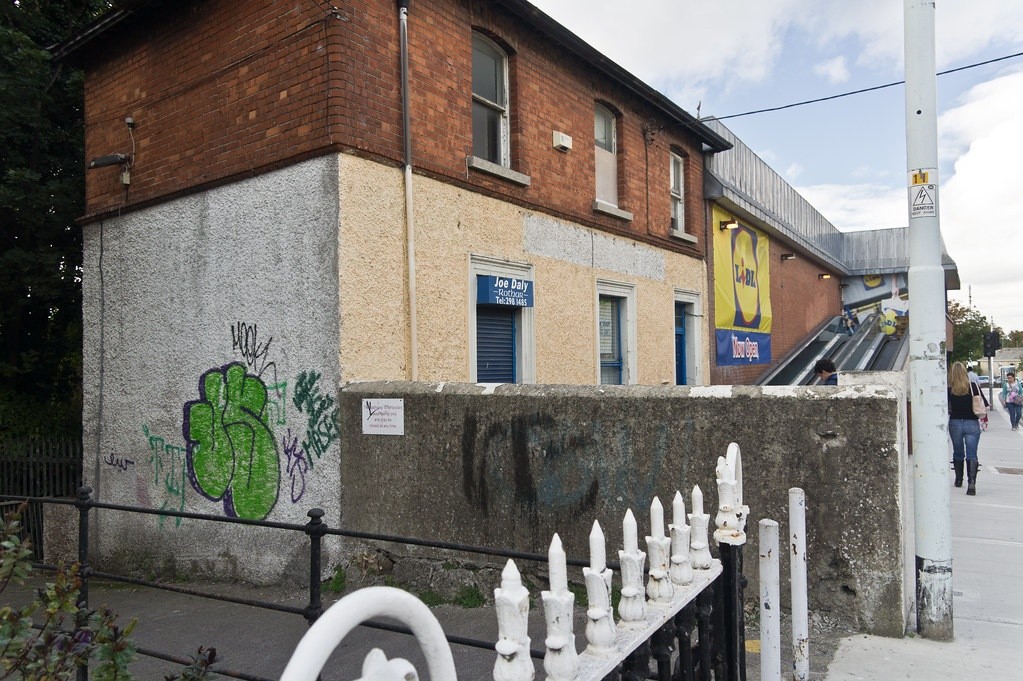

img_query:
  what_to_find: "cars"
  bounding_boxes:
[994,375,1021,387]
[978,376,991,387]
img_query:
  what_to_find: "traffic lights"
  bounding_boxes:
[983,331,995,357]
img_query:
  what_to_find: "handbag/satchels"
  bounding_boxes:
[970,380,987,419]
[1014,394,1023,407]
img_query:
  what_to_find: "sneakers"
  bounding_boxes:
[1012,423,1019,431]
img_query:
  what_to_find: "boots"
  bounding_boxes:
[950,458,982,495]
